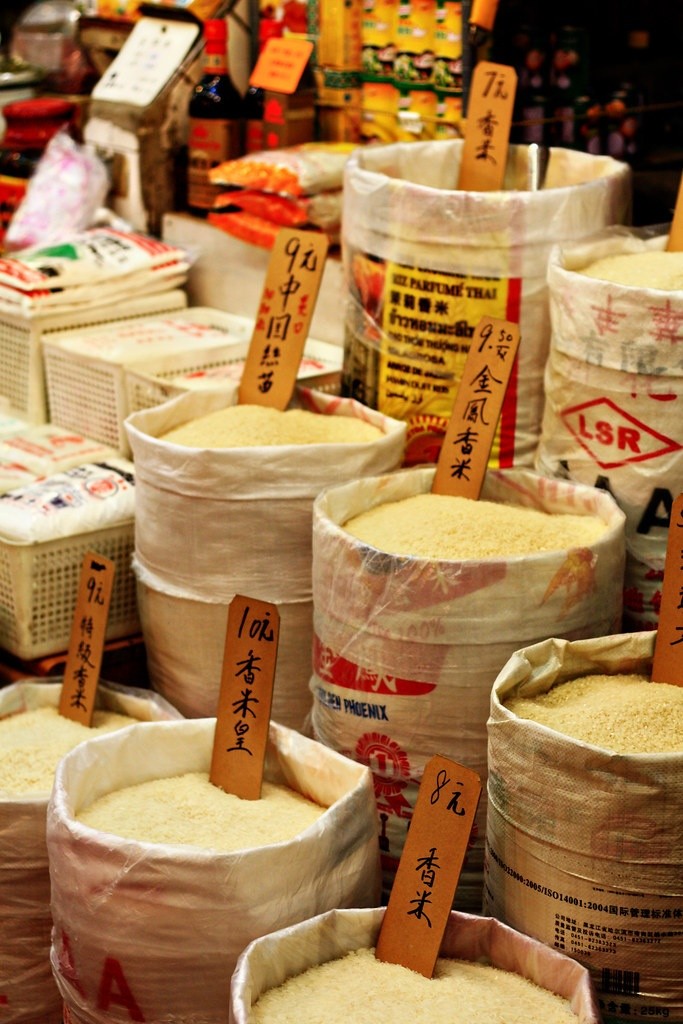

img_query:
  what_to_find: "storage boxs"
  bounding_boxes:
[0,291,342,661]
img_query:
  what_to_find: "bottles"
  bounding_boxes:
[243,20,281,153]
[512,22,654,167]
[260,4,317,150]
[187,19,242,216]
